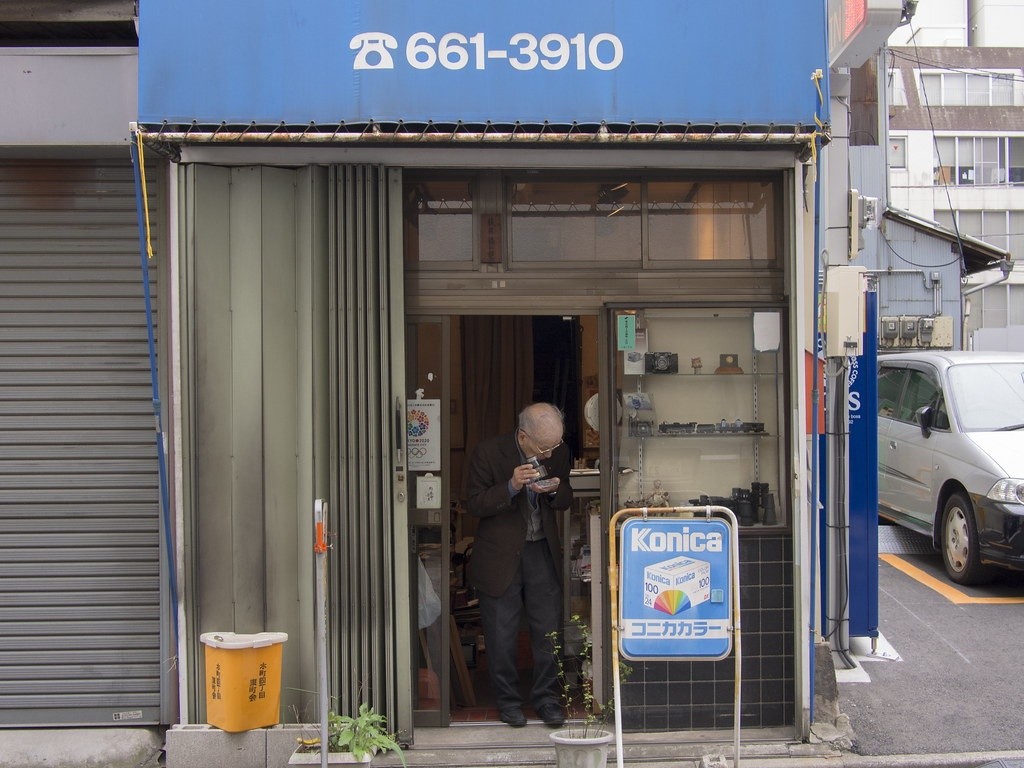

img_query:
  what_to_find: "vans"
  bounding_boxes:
[877,351,1024,586]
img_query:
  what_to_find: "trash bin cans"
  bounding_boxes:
[199,630,288,732]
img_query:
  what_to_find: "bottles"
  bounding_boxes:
[526,456,548,478]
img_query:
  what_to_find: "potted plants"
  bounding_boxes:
[544,615,633,768]
[287,701,407,768]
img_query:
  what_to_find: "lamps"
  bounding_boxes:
[597,180,629,217]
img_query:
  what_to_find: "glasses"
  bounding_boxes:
[520,428,564,455]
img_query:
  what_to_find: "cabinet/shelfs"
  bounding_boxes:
[600,301,793,536]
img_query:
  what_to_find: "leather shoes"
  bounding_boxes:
[537,703,564,725]
[500,707,526,727]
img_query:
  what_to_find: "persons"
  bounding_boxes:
[464,401,576,728]
[643,479,670,517]
